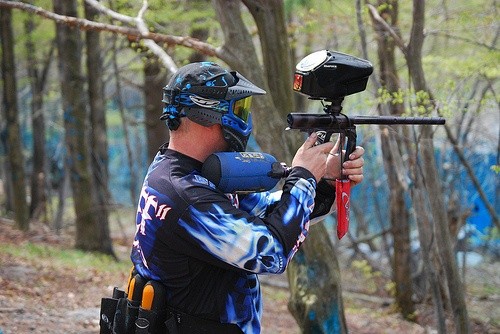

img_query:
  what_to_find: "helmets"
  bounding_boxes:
[159,62,268,152]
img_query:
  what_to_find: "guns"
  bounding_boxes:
[201,49,447,240]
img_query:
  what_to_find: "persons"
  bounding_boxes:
[130,61,365,334]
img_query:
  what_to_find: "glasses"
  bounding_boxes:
[229,95,252,123]
